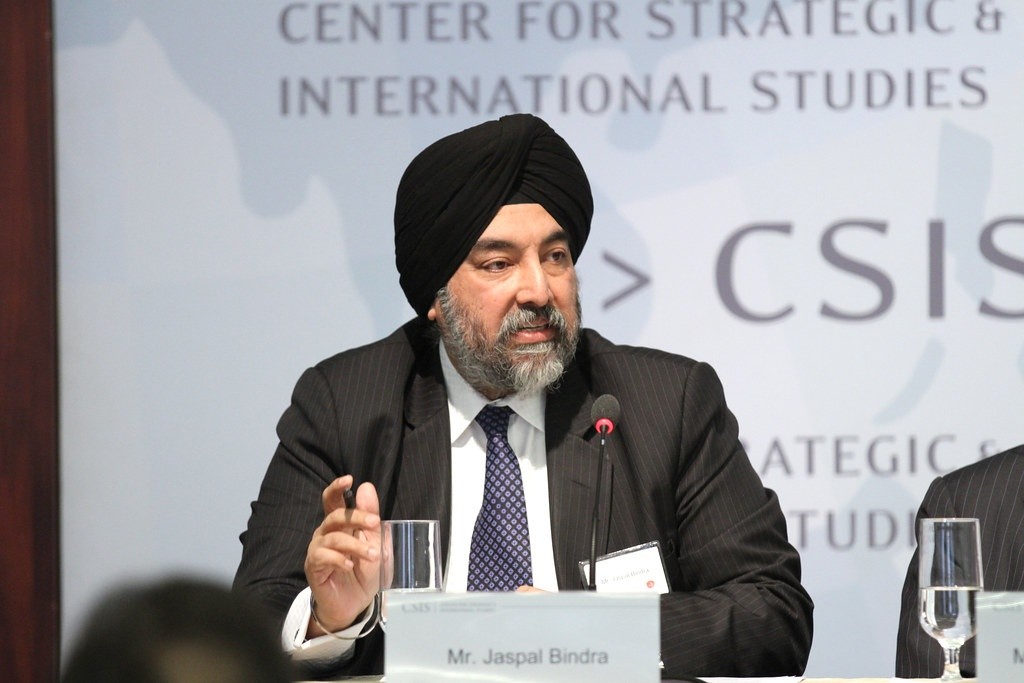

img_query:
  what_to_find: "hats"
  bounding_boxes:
[394,114,594,323]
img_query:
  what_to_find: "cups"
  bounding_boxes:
[377,519,442,629]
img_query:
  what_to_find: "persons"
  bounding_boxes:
[895,442,1024,678]
[60,570,297,682]
[231,113,815,682]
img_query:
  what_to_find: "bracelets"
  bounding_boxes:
[310,589,380,639]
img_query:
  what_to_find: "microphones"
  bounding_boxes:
[587,394,621,590]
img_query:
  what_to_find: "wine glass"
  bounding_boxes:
[919,519,984,681]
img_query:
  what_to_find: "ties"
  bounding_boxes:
[467,403,532,592]
